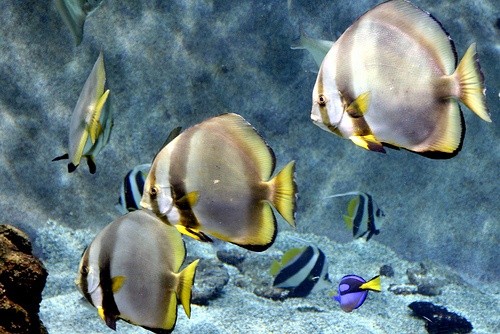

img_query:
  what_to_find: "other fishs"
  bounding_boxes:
[51,51,114,175]
[330,274,382,313]
[310,0,492,160]
[138,111,299,253]
[290,16,336,68]
[72,211,201,334]
[326,191,387,242]
[267,234,332,298]
[112,163,153,214]
